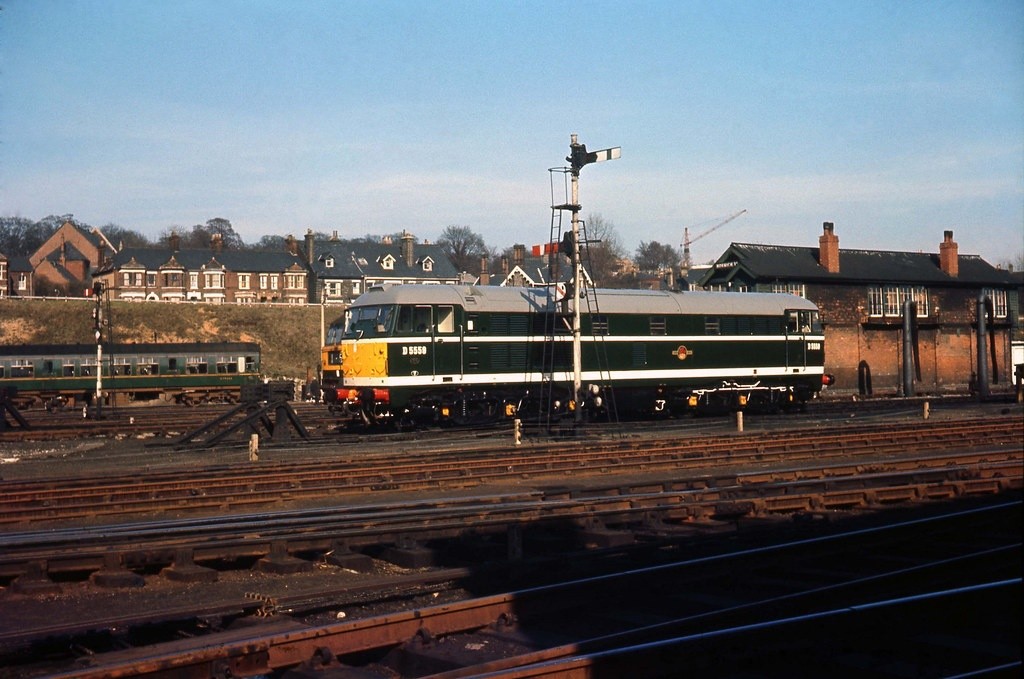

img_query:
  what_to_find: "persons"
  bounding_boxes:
[82,404,89,418]
[310,376,322,403]
[44,400,51,412]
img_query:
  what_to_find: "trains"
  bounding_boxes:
[1,341,262,405]
[318,283,836,437]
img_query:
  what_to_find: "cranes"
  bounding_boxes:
[683,209,748,266]
